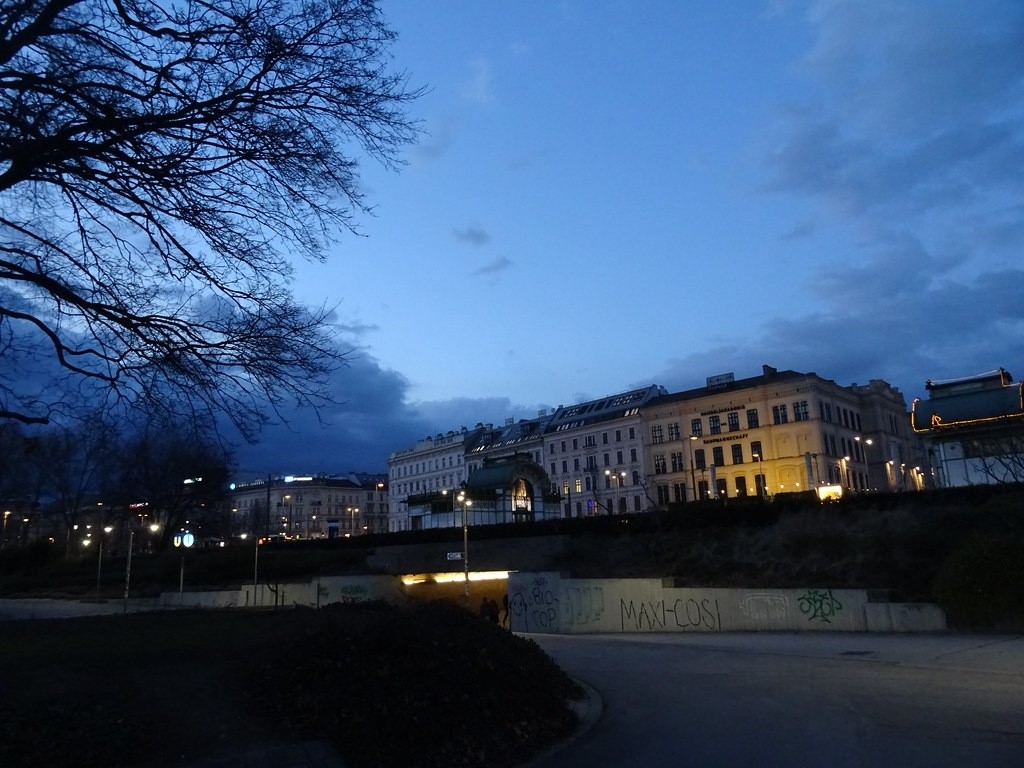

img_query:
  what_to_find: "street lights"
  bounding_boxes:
[307,514,317,540]
[457,491,473,582]
[752,453,766,502]
[374,481,384,535]
[604,470,627,501]
[103,524,160,599]
[854,436,873,495]
[282,495,290,522]
[172,531,195,593]
[688,434,698,501]
[240,533,259,607]
[347,507,359,538]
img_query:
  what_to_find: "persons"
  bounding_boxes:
[481,595,509,626]
